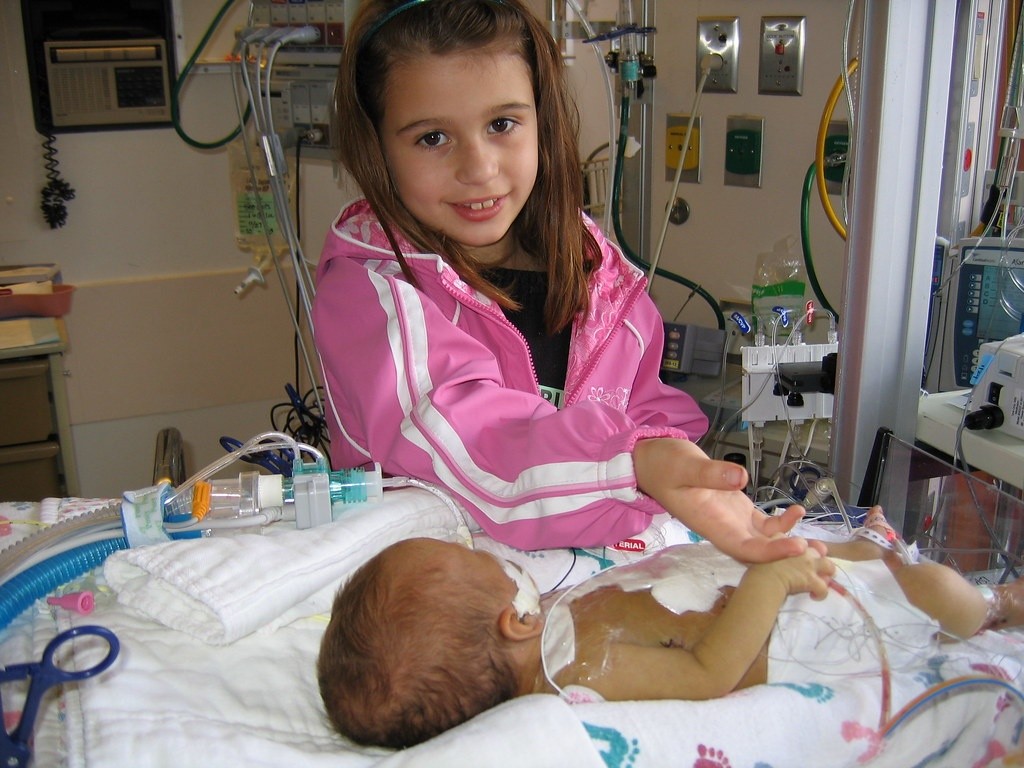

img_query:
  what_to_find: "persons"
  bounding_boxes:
[317,506,1024,750]
[312,0,827,567]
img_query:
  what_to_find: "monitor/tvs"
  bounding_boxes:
[919,236,1024,394]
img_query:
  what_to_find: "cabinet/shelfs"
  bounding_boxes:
[0,316,82,500]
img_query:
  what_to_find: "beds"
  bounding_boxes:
[0,492,1024,768]
